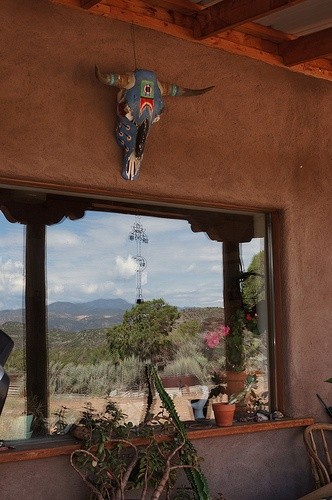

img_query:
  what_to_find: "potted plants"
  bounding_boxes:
[212,374,257,427]
[206,303,263,412]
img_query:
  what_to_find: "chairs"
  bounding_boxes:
[303,422,332,490]
